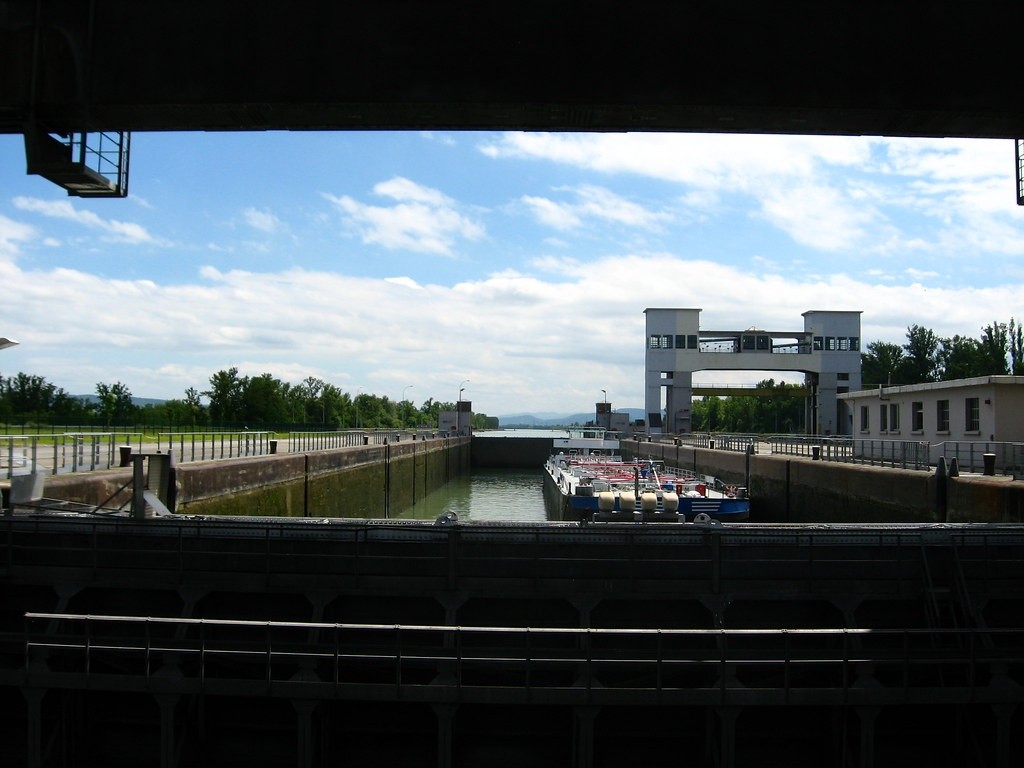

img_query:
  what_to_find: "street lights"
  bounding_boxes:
[602,389,607,414]
[402,385,413,402]
[459,379,471,401]
